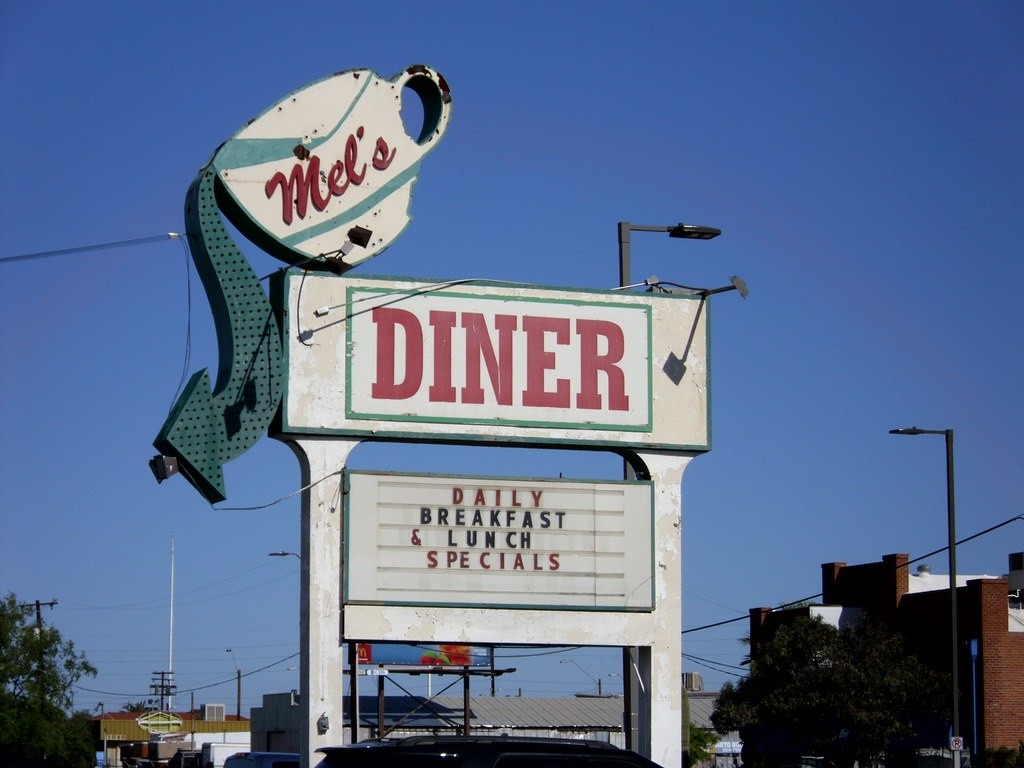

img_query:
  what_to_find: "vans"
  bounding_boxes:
[223,750,300,768]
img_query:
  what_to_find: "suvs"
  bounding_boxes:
[313,733,662,768]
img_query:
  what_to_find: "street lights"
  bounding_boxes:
[617,222,719,290]
[887,425,964,768]
[225,647,242,722]
